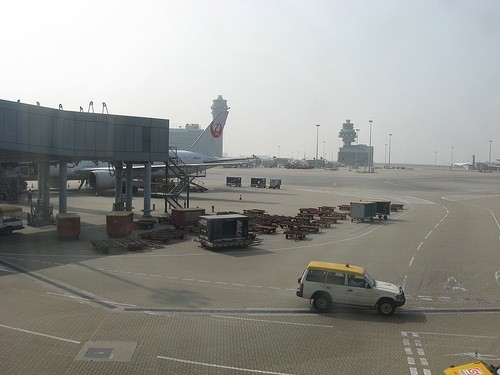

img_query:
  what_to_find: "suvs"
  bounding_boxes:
[296,260,405,317]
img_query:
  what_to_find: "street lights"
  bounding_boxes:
[489,140,492,162]
[356,129,360,168]
[384,143,387,164]
[388,133,392,166]
[452,147,454,165]
[369,120,374,173]
[435,151,437,167]
[316,124,320,159]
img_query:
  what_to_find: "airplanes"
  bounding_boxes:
[50,107,260,195]
